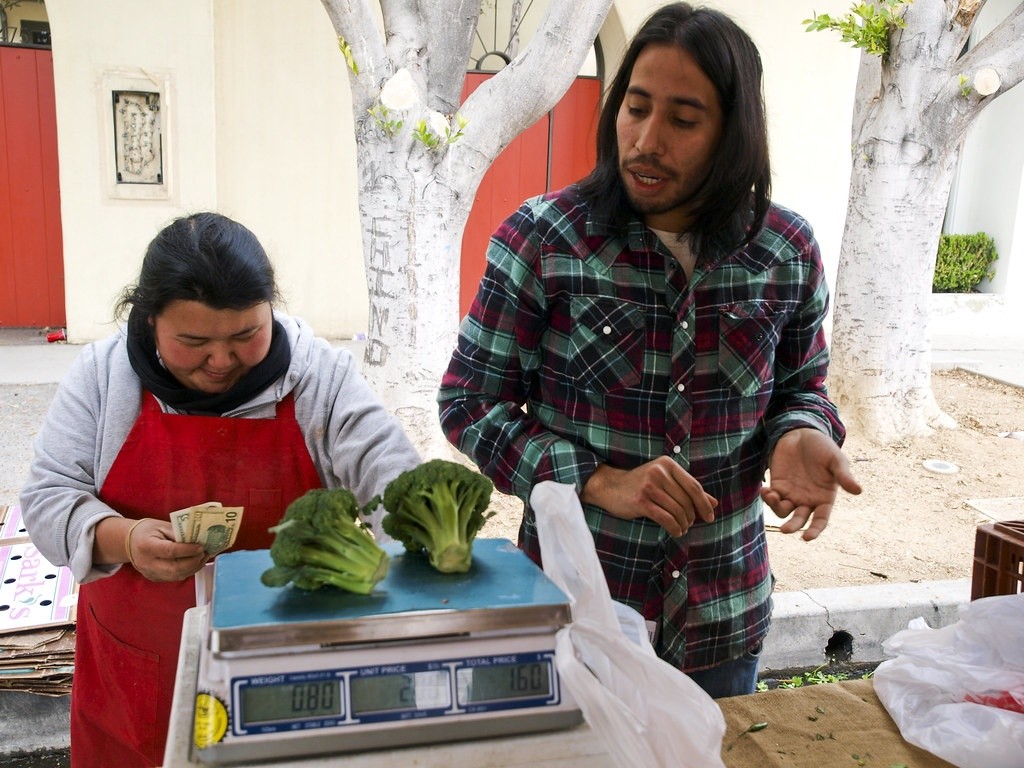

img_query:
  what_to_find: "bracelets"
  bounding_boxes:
[126,517,157,573]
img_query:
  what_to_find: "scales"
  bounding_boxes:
[189,540,584,765]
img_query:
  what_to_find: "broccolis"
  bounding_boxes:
[383,459,497,572]
[260,486,390,594]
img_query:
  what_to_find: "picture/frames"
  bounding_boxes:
[102,69,173,200]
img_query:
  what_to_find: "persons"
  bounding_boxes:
[18,210,429,768]
[439,1,862,696]
